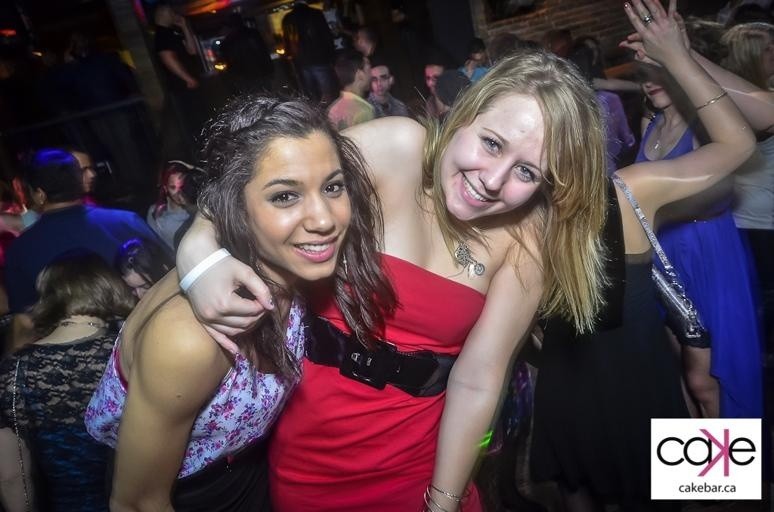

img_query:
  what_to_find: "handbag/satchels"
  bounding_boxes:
[650,263,706,341]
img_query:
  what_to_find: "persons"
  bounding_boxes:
[0,0,774,512]
